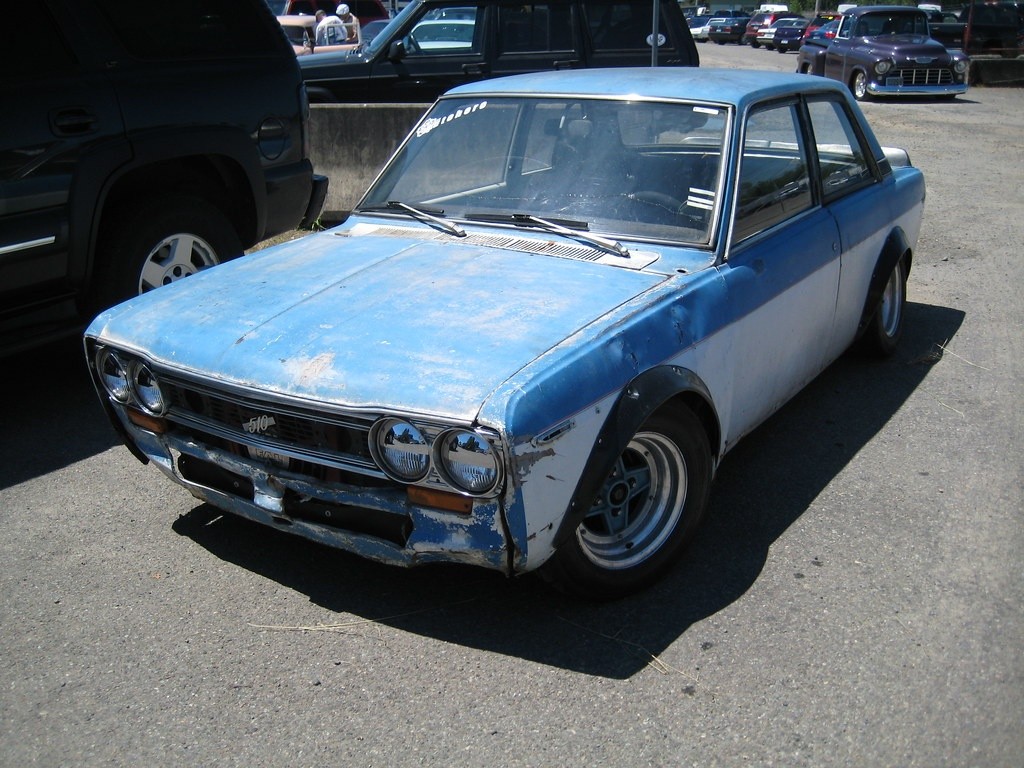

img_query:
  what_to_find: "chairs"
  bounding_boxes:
[879,20,899,34]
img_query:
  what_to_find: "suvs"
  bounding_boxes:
[745,11,805,48]
[0,0,331,302]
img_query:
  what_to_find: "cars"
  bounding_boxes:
[273,14,361,56]
[773,20,812,54]
[265,0,388,37]
[417,7,478,20]
[360,18,392,42]
[795,6,970,101]
[401,18,475,47]
[681,7,753,46]
[923,0,1024,84]
[294,0,702,103]
[809,19,857,42]
[83,63,926,590]
[799,12,845,46]
[756,17,800,50]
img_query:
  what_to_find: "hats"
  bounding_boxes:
[336,3,350,16]
[315,9,325,22]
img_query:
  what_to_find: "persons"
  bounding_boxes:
[316,3,360,46]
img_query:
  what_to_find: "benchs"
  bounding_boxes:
[619,151,806,202]
